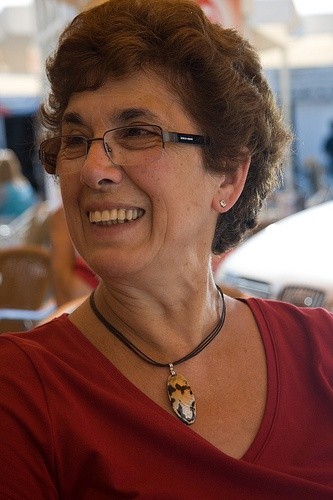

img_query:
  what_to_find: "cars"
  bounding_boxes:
[214,199,333,315]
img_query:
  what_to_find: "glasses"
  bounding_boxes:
[39,125,210,175]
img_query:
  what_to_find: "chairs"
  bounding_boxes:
[0,245,51,331]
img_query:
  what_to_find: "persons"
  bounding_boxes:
[0,149,34,223]
[0,0,333,500]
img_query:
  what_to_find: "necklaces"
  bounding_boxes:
[89,280,226,428]
